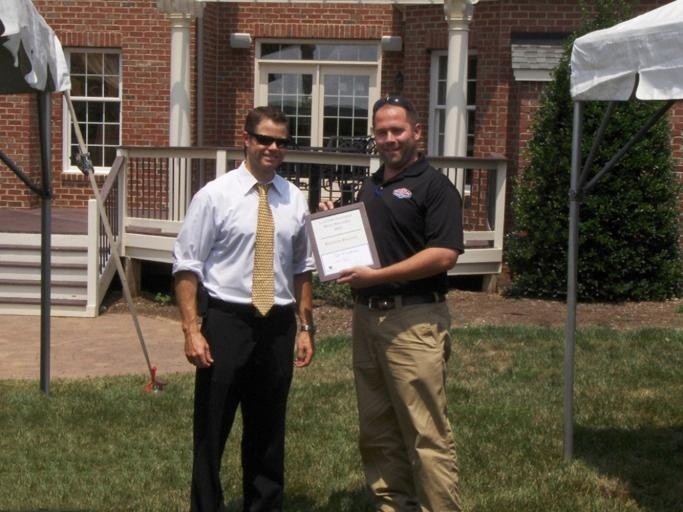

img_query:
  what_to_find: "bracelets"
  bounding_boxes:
[301,324,316,332]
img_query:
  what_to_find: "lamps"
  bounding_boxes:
[231,32,253,48]
[381,35,402,52]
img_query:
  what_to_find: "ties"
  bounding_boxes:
[252,183,275,317]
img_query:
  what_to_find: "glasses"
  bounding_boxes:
[374,96,405,110]
[248,132,290,148]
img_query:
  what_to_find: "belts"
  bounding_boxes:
[352,292,446,310]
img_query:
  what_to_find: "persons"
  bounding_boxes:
[316,96,464,512]
[172,106,314,512]
